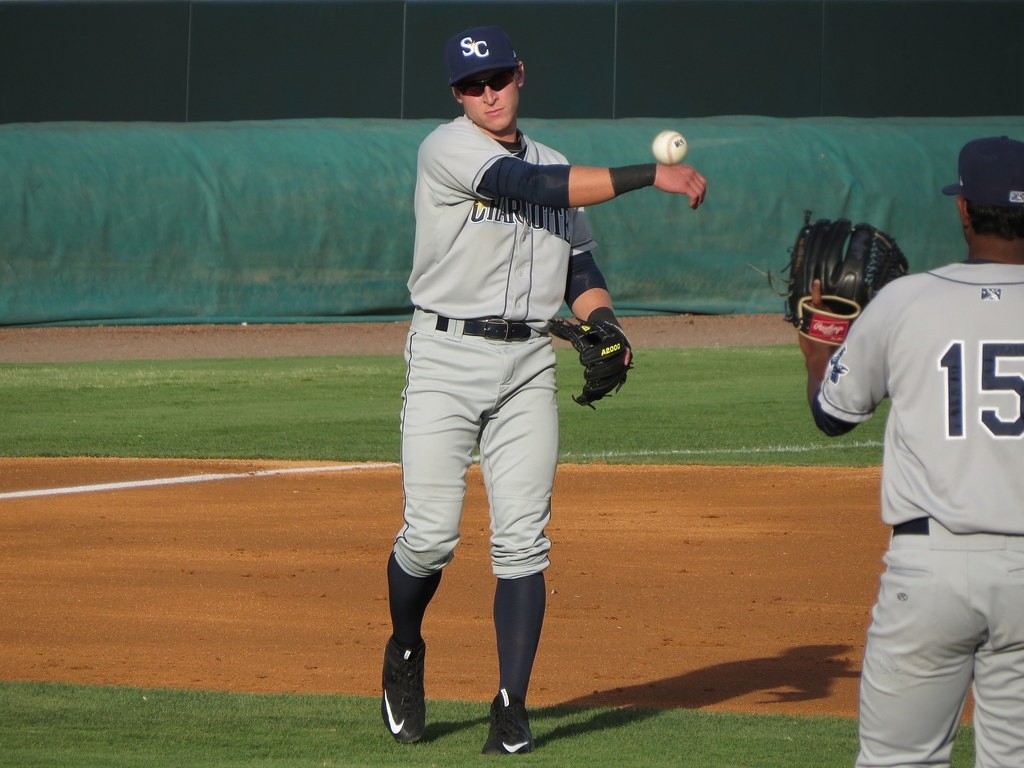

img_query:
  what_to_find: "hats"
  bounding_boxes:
[444,26,519,88]
[940,135,1024,196]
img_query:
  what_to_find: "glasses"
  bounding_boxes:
[451,65,519,97]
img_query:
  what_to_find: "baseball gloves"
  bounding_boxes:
[547,316,635,408]
[780,216,911,347]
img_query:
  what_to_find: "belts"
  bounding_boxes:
[892,516,929,536]
[436,316,530,341]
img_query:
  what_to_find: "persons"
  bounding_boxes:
[381,27,706,755]
[799,135,1024,768]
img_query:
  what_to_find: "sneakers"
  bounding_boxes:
[483,689,534,754]
[381,634,426,745]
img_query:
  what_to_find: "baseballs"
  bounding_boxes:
[650,129,690,166]
